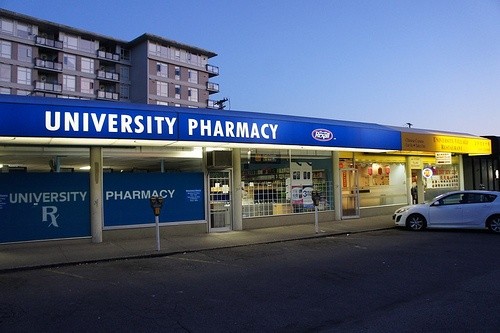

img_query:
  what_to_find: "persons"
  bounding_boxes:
[411,182,418,204]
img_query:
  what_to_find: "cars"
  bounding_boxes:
[392,190,500,234]
